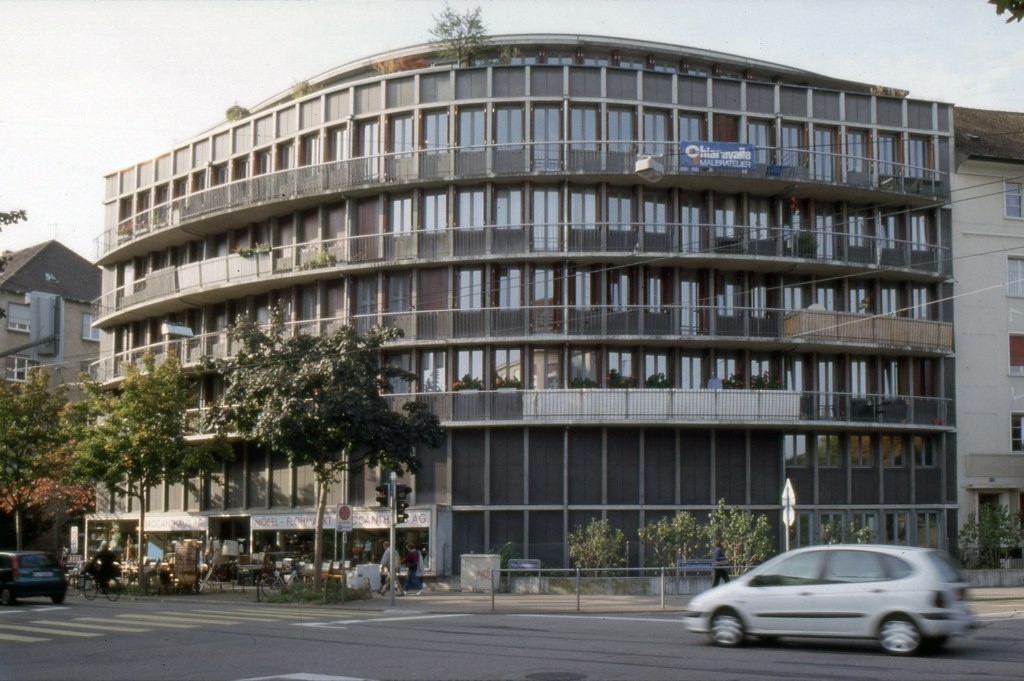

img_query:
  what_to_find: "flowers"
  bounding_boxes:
[723,371,780,391]
[117,220,147,235]
[494,374,521,388]
[452,374,484,391]
[606,368,634,388]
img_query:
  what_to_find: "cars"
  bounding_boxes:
[682,542,983,656]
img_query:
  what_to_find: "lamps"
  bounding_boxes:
[634,153,666,184]
[160,321,195,339]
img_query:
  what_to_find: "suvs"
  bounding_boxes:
[0,550,69,606]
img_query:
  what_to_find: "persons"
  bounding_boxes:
[711,539,729,587]
[401,542,423,595]
[379,541,405,596]
[91,541,115,593]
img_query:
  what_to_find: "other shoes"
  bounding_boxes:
[417,590,424,596]
[378,591,384,596]
[395,592,403,596]
[404,591,406,595]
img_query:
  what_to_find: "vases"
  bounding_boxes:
[498,387,517,393]
[117,229,149,242]
[459,389,480,394]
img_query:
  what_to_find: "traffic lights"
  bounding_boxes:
[396,483,413,523]
[375,483,390,507]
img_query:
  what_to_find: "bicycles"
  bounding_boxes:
[78,561,122,602]
[260,565,305,603]
